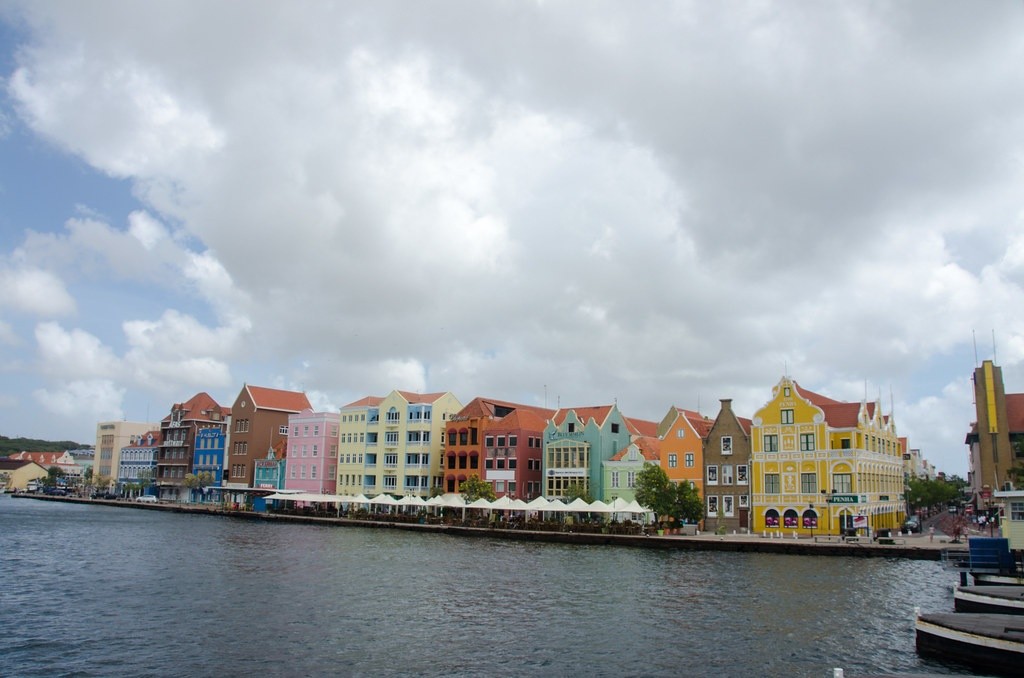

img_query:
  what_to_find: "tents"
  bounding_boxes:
[263,491,652,534]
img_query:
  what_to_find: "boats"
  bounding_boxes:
[915,612,1024,662]
[954,585,1024,614]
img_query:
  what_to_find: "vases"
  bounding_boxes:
[821,489,827,494]
[832,489,837,493]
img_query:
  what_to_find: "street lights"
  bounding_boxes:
[809,504,814,538]
[989,514,995,538]
[821,488,837,540]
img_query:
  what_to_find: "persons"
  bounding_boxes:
[929,528,934,543]
[977,513,987,531]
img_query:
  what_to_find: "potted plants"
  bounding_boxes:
[675,480,704,536]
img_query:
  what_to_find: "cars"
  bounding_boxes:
[46,488,66,496]
[239,502,251,511]
[842,528,859,541]
[905,516,922,530]
[224,503,237,511]
[136,495,159,504]
[948,506,956,512]
[901,521,920,534]
